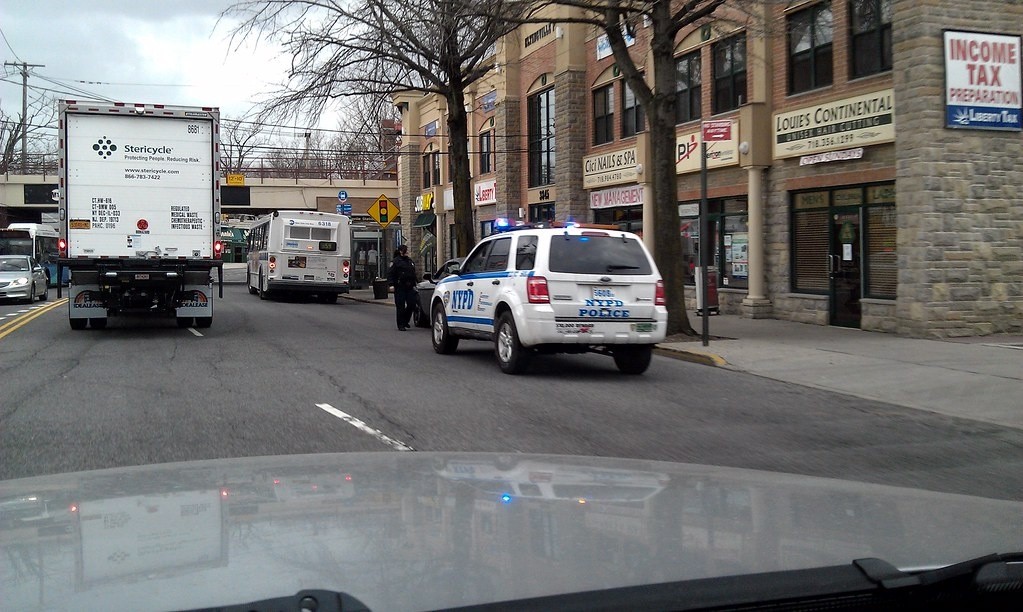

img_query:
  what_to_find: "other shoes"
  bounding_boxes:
[399,324,410,331]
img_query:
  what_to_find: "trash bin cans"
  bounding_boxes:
[373,278,388,299]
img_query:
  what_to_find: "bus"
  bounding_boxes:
[0,223,70,287]
[243,208,352,306]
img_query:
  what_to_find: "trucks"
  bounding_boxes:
[56,98,225,330]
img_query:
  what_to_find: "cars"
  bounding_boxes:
[413,256,467,328]
[0,255,49,303]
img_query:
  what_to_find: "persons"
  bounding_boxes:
[367,244,378,280]
[388,244,419,331]
[356,246,368,279]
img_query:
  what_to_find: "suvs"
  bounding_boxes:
[428,215,669,376]
